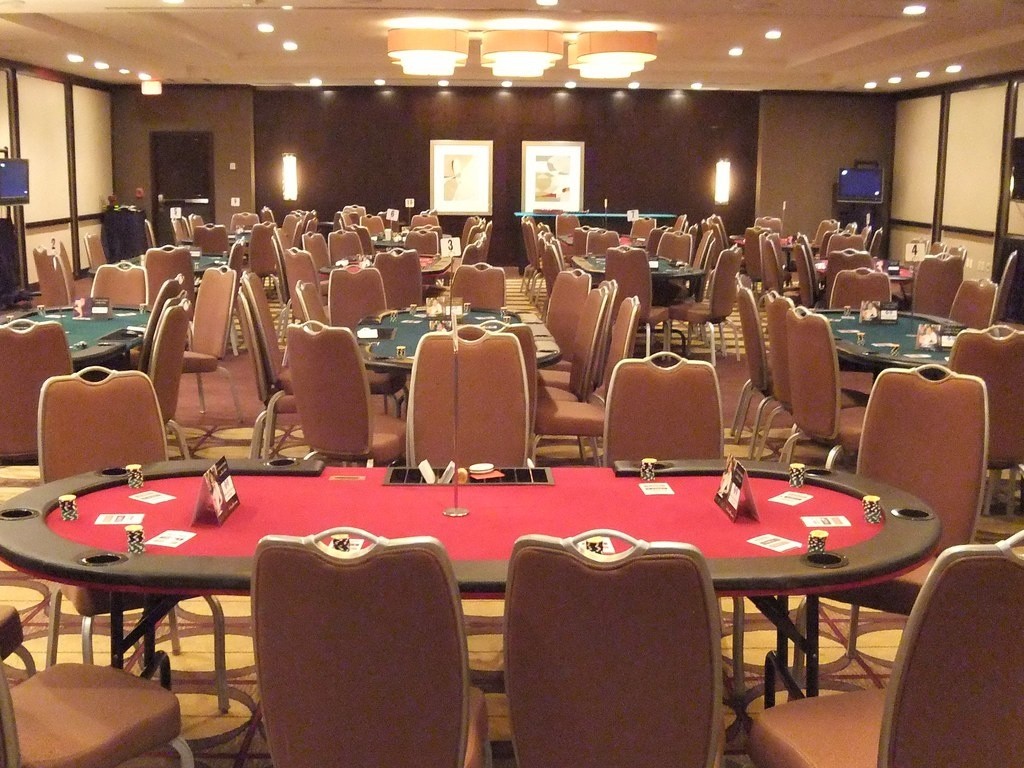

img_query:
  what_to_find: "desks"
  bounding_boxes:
[100,210,148,263]
[789,255,915,303]
[87,254,229,279]
[513,213,677,218]
[180,231,251,261]
[319,254,454,274]
[0,304,152,371]
[728,235,821,271]
[369,240,403,248]
[0,459,941,710]
[795,306,966,387]
[354,304,565,374]
[570,250,706,356]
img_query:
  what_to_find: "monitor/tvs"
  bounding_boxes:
[837,167,884,203]
[0,158,30,205]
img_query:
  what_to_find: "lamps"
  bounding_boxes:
[716,157,731,203]
[385,27,658,80]
[141,80,160,96]
[283,153,298,201]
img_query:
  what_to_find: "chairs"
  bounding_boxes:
[0,203,1024,768]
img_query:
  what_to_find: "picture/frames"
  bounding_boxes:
[522,141,585,213]
[430,140,493,216]
